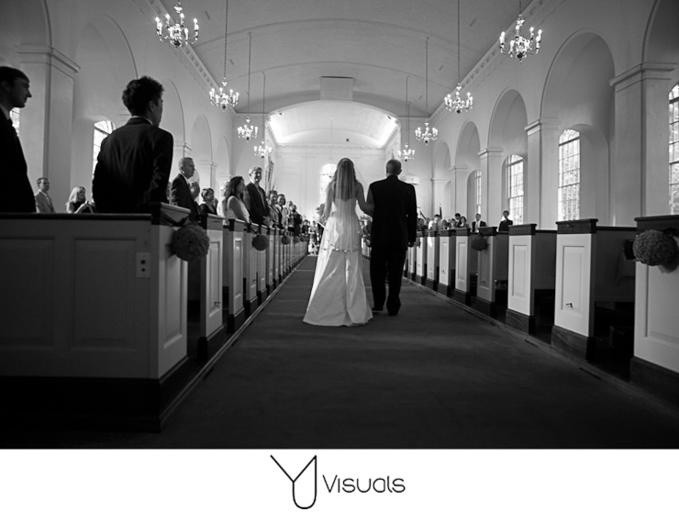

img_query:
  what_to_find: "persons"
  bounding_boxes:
[458,216,468,228]
[308,220,317,254]
[441,219,451,229]
[226,177,253,225]
[451,218,459,228]
[276,194,285,227]
[213,198,218,208]
[428,214,441,234]
[360,221,366,235]
[199,188,217,226]
[284,200,303,236]
[367,160,417,316]
[418,218,428,230]
[301,220,312,254]
[35,177,54,213]
[92,76,173,225]
[246,166,269,225]
[498,211,513,232]
[222,180,230,222]
[170,156,200,211]
[472,214,486,233]
[66,185,93,214]
[190,182,200,199]
[302,158,375,326]
[365,220,372,236]
[267,190,284,229]
[455,213,461,226]
[0,66,38,214]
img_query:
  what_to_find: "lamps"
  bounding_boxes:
[397,76,415,162]
[414,39,439,144]
[238,33,259,141]
[208,0,240,111]
[498,0,543,62]
[444,1,474,114]
[255,72,272,160]
[154,0,201,49]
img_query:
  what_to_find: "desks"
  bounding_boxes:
[506,224,558,337]
[245,220,261,311]
[267,227,277,294]
[427,229,439,290]
[416,232,426,284]
[408,245,416,281]
[188,213,226,345]
[438,229,455,297]
[223,217,248,325]
[0,210,187,383]
[629,214,679,405]
[274,227,282,288]
[551,218,634,360]
[259,223,269,301]
[454,226,478,308]
[474,227,508,317]
